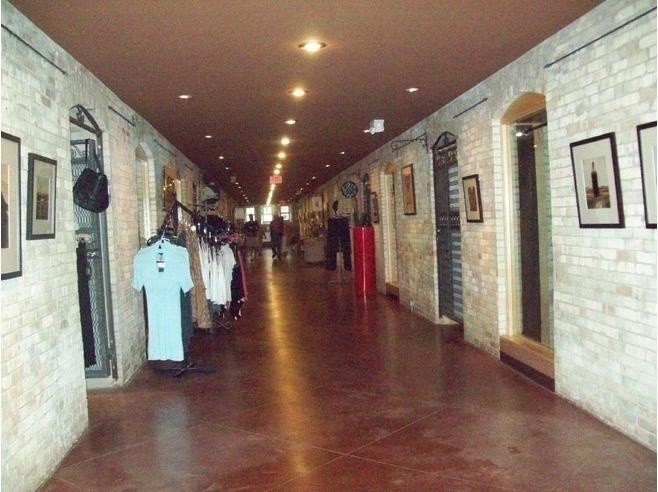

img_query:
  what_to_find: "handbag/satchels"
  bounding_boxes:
[73,169,108,212]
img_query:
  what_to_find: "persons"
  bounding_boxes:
[270,211,284,260]
[244,213,261,261]
[254,218,268,256]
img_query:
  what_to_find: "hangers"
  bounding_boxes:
[144,202,230,254]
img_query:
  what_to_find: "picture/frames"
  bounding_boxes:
[1,131,57,281]
[370,192,380,223]
[568,119,657,229]
[462,174,484,223]
[400,163,416,215]
